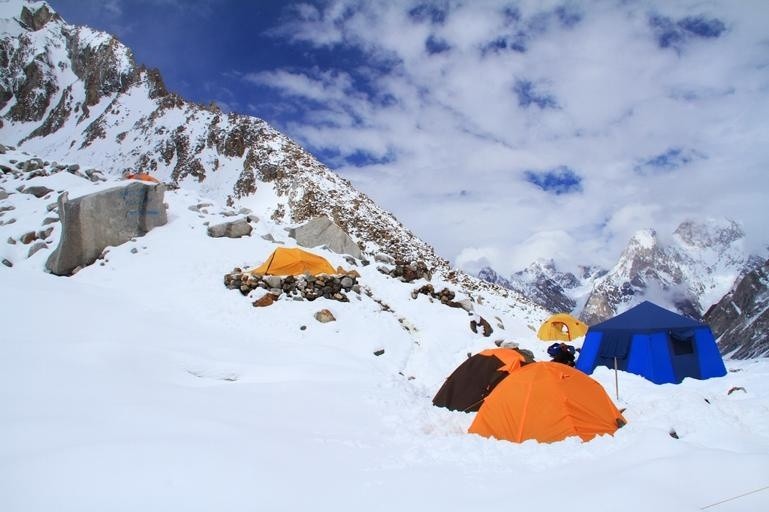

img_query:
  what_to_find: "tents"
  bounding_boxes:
[243,245,338,277]
[468,359,629,444]
[575,300,728,385]
[431,347,537,413]
[536,312,589,341]
[127,172,160,184]
